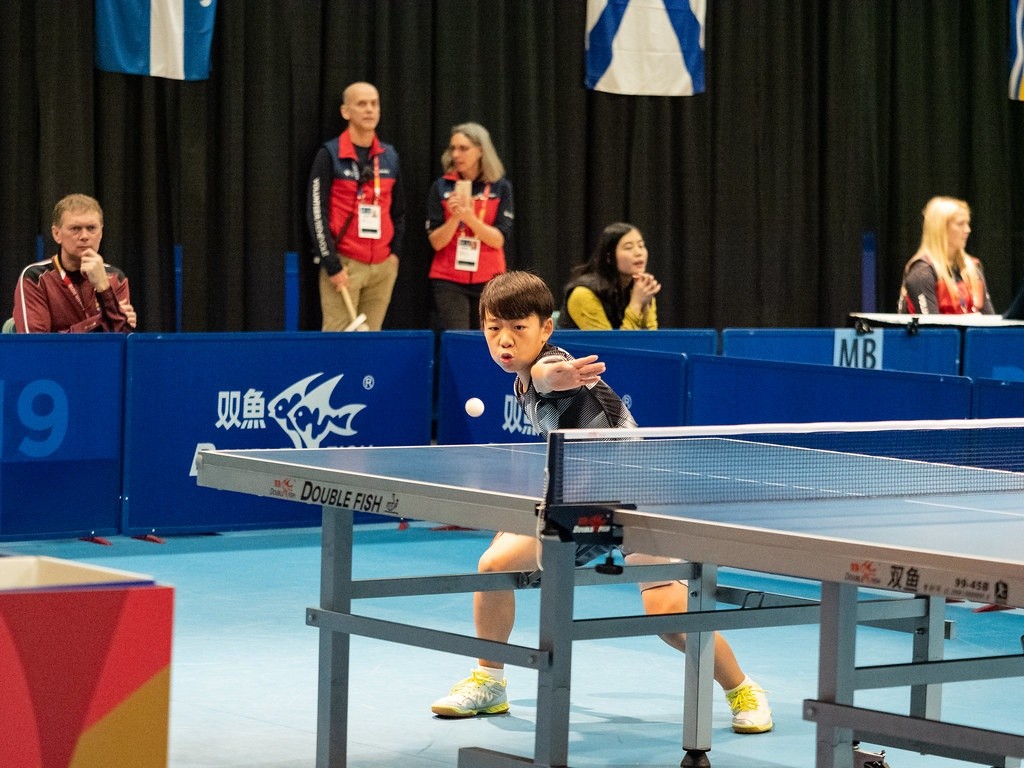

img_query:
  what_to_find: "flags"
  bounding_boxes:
[1010,0,1024,101]
[586,0,707,96]
[95,0,216,80]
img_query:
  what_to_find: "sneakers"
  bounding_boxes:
[723,681,773,732]
[432,669,509,716]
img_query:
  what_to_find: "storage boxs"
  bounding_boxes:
[0,549,177,767]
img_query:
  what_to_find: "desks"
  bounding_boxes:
[196,419,1024,768]
[850,311,1023,330]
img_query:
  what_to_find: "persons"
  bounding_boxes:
[307,82,405,329]
[556,224,661,331]
[12,195,135,330]
[898,197,994,314]
[429,124,513,327]
[432,272,773,734]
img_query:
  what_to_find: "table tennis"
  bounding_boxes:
[465,397,486,418]
